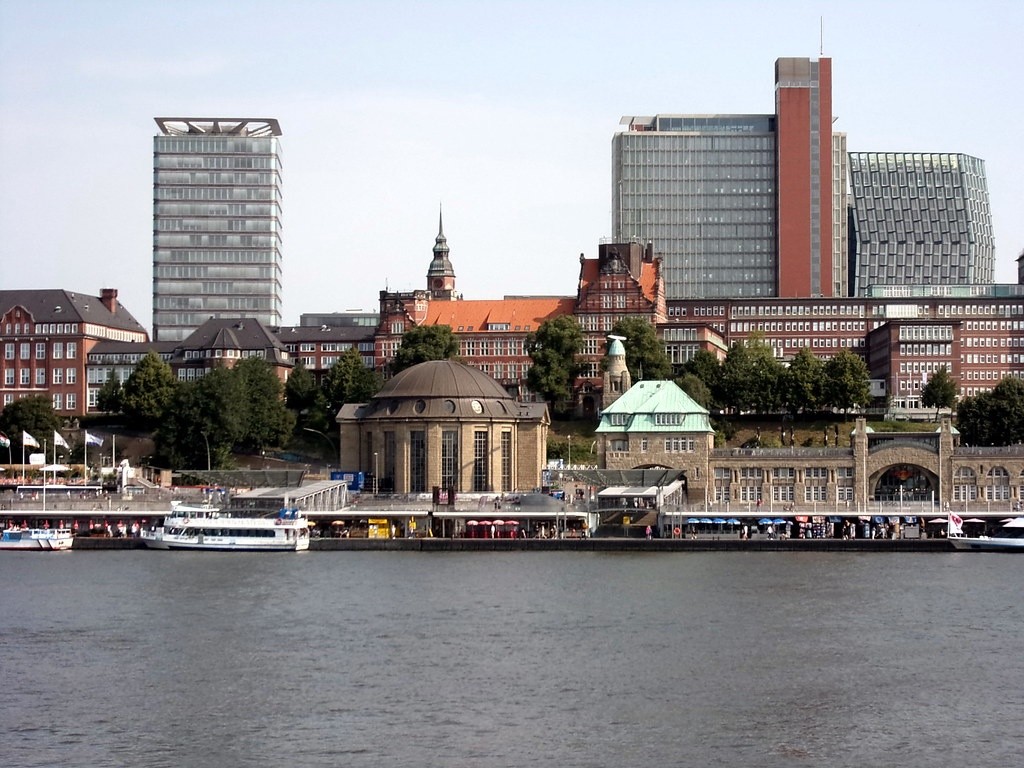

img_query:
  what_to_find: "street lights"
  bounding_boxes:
[302,427,339,477]
[565,434,574,471]
[200,431,213,485]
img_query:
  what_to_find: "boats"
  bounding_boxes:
[140,504,312,553]
[0,523,74,551]
[949,505,1024,553]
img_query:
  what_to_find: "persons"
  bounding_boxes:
[1,474,223,505]
[560,489,926,540]
[101,523,140,537]
[493,500,502,513]
[311,521,588,539]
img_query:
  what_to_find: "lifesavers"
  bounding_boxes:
[275,519,282,525]
[182,517,190,524]
[673,527,680,535]
